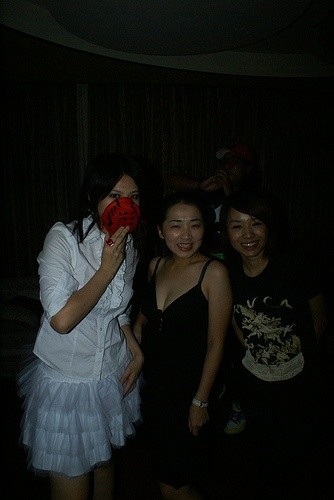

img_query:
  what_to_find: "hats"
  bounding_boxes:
[223,143,256,165]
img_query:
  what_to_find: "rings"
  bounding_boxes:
[108,239,115,247]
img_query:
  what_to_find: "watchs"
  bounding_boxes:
[193,399,208,407]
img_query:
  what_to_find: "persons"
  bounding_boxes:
[133,189,234,500]
[19,162,144,500]
[168,150,260,259]
[221,190,334,500]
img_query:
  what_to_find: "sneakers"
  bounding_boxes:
[224,400,247,435]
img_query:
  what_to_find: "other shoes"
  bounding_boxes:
[214,383,228,400]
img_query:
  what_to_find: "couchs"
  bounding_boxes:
[0,278,43,382]
